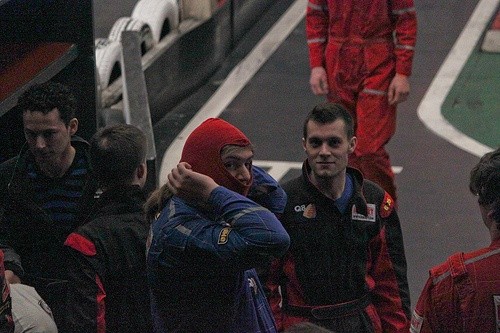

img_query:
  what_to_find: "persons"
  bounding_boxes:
[259,104,411,333]
[408,149,500,332]
[306,0,417,208]
[0,82,154,333]
[145,118,291,333]
